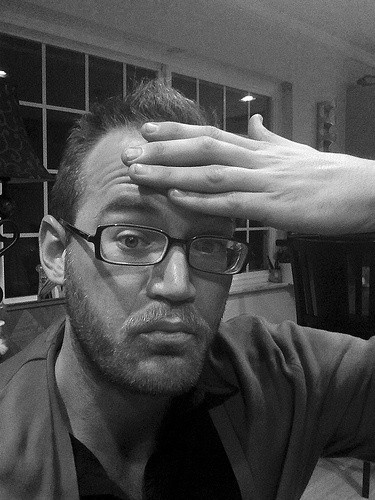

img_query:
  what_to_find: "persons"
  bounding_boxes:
[0,73,375,500]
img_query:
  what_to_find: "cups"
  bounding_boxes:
[269,269,281,282]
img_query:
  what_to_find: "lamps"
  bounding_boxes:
[0,79,55,304]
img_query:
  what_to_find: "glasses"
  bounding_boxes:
[55,217,255,276]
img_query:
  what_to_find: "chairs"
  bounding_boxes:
[275,229,375,500]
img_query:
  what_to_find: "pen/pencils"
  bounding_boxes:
[267,256,282,270]
